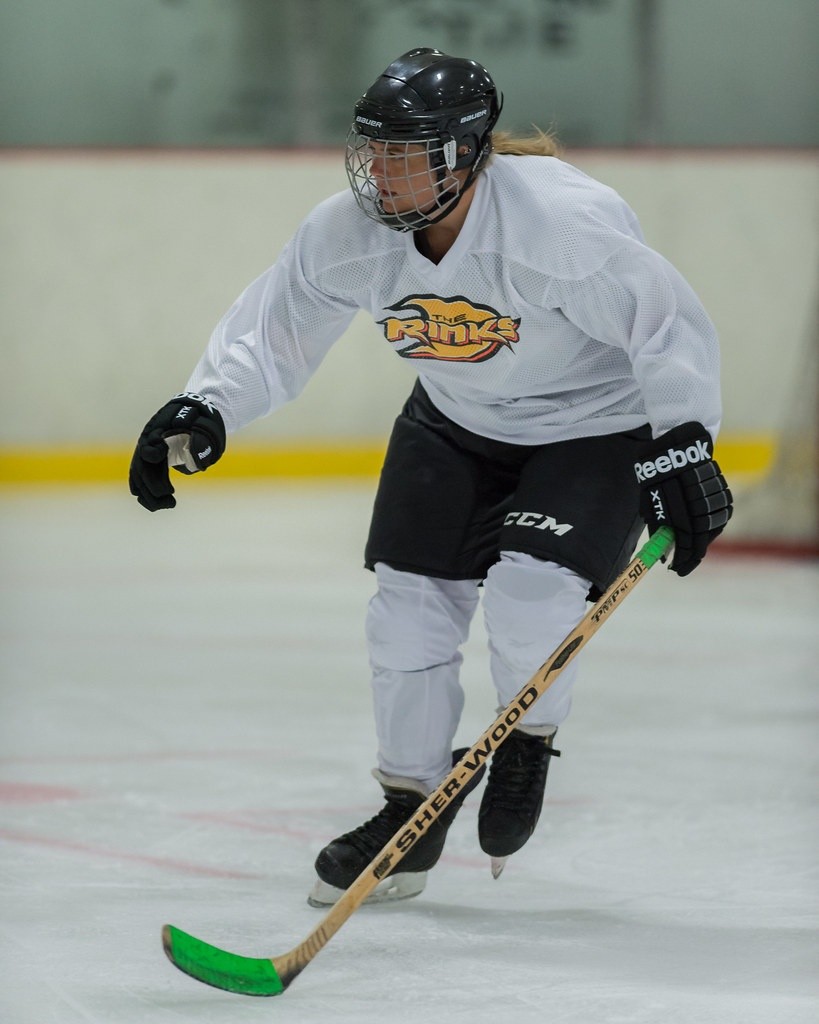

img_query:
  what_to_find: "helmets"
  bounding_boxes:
[344,48,505,234]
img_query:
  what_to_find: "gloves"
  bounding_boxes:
[634,422,735,577]
[128,392,226,512]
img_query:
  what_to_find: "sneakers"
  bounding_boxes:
[477,706,562,880]
[307,747,487,910]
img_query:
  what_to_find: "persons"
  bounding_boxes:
[126,44,738,911]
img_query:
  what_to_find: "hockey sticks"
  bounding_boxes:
[159,525,676,998]
